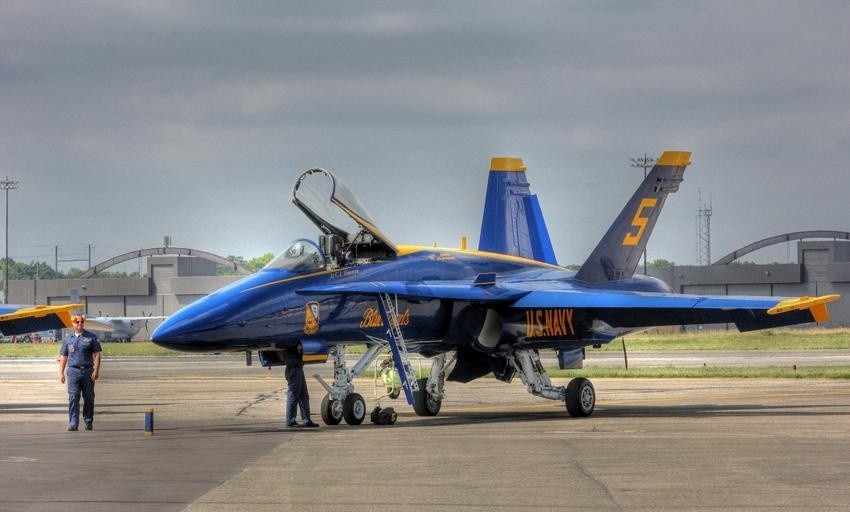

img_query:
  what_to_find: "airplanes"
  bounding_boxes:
[85,310,170,343]
[147,149,837,426]
[0,304,85,336]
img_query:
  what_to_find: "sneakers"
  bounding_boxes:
[67,425,78,431]
[84,423,93,430]
[286,420,304,427]
[303,421,320,428]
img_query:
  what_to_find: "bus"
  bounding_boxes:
[0,328,62,342]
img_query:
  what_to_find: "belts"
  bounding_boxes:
[286,364,304,369]
[70,365,95,370]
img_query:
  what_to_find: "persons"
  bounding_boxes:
[58,315,101,431]
[283,337,322,427]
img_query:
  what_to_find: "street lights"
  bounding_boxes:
[0,176,23,305]
[629,153,660,276]
